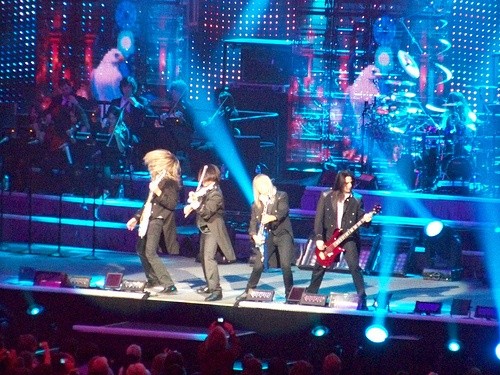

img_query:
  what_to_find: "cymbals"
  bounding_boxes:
[397,49,421,80]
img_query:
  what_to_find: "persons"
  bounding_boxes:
[184,164,236,301]
[437,92,477,153]
[0,319,481,375]
[305,170,373,306]
[235,174,296,304]
[318,106,351,136]
[127,149,182,295]
[44,79,241,182]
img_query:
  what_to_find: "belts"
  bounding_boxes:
[153,218,164,221]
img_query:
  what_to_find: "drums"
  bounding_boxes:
[441,152,475,180]
[395,154,426,190]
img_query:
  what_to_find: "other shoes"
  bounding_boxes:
[286,293,294,304]
[197,285,210,294]
[158,285,177,294]
[358,299,366,310]
[205,295,222,301]
[144,282,152,288]
[236,291,248,301]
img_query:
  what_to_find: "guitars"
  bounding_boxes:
[137,168,166,238]
[314,201,383,268]
[255,194,271,262]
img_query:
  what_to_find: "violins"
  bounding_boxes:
[187,186,208,203]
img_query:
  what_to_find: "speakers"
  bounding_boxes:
[226,44,295,179]
